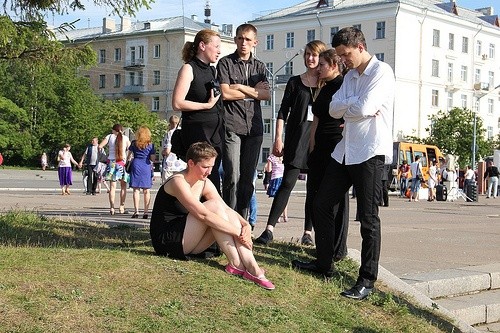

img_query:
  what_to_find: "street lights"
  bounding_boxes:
[472,84,500,171]
[252,48,305,145]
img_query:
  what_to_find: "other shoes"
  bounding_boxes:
[255,229,273,244]
[301,233,314,246]
[225,262,264,275]
[83,189,110,195]
[243,269,275,290]
[62,190,70,196]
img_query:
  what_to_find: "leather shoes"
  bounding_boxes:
[339,284,375,300]
[291,258,335,277]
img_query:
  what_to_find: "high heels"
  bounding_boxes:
[110,208,116,215]
[119,206,125,214]
[143,214,148,219]
[131,214,139,219]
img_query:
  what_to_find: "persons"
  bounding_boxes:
[288,26,395,301]
[0,23,500,261]
[150,142,275,290]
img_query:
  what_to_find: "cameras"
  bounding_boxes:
[206,80,221,97]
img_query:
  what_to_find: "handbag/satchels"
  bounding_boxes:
[93,162,108,175]
[168,114,212,164]
[416,163,423,180]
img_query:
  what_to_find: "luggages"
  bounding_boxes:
[436,177,447,201]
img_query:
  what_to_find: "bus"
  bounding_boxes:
[386,141,446,191]
